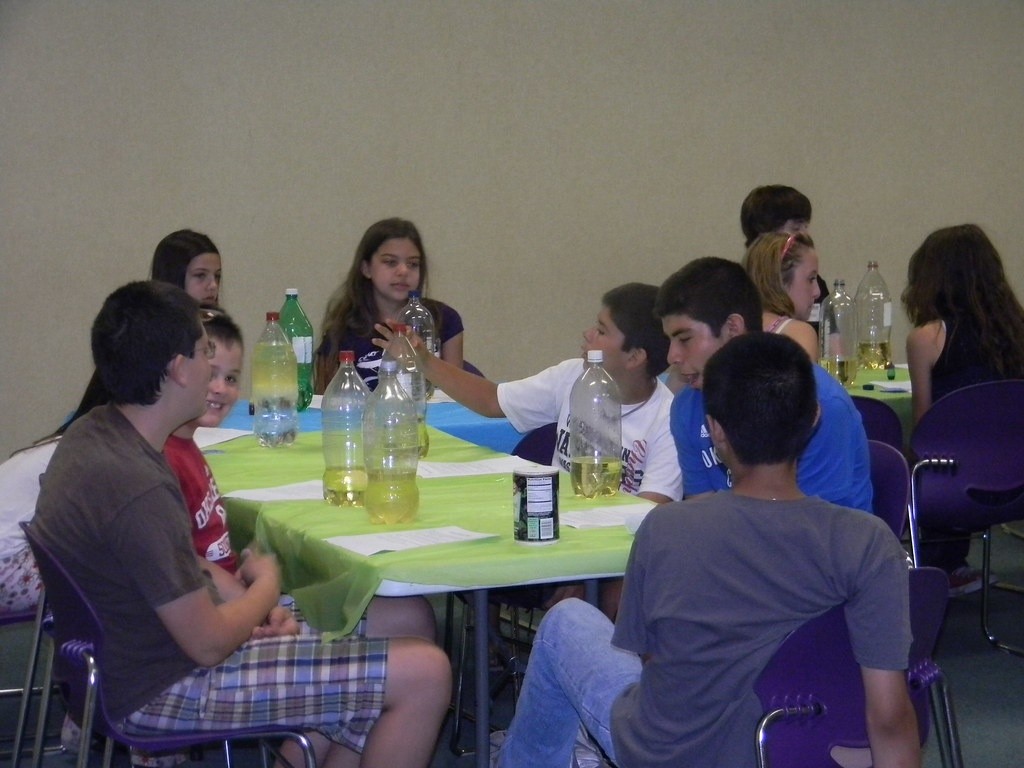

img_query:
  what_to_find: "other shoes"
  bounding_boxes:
[947,566,998,597]
[487,634,504,668]
[489,730,508,768]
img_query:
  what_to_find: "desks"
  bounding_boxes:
[195,417,658,768]
[830,367,916,455]
[215,396,524,447]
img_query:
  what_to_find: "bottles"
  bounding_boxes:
[854,261,894,372]
[379,327,431,459]
[276,287,314,413]
[250,312,301,448]
[361,360,421,526]
[400,289,438,401]
[320,350,371,509]
[567,349,626,500]
[817,278,859,389]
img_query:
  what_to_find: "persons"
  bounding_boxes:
[742,228,822,364]
[370,283,684,676]
[901,223,1024,594]
[161,302,328,768]
[599,256,874,626]
[56,226,228,426]
[478,327,923,767]
[739,184,836,344]
[0,428,189,768]
[23,280,455,768]
[310,215,485,394]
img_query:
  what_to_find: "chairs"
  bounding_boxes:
[0,364,1022,768]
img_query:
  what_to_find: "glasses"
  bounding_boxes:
[199,307,222,322]
